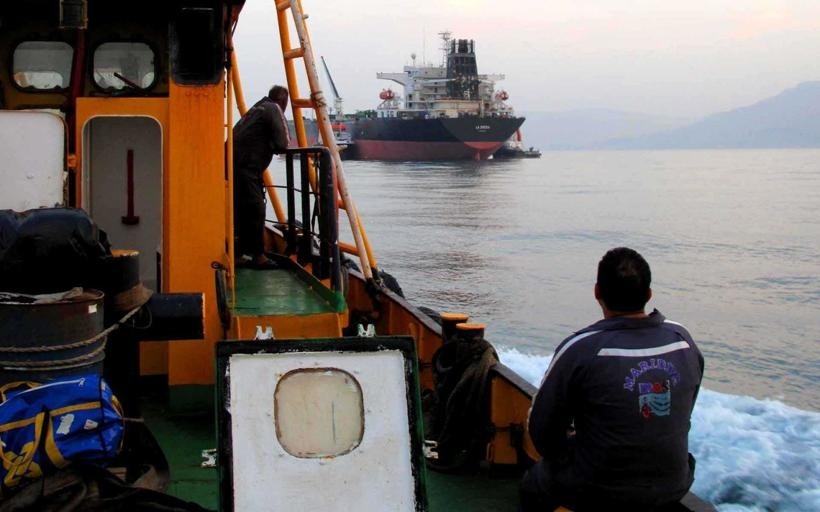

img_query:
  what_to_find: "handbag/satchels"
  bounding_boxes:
[0,207,116,296]
[0,373,128,501]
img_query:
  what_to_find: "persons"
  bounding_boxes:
[225,85,292,270]
[516,247,704,512]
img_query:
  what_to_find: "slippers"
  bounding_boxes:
[254,259,280,270]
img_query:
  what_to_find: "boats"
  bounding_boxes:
[493,126,542,159]
[279,55,355,158]
[3,0,723,511]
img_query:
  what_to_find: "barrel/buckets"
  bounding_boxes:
[0,289,106,384]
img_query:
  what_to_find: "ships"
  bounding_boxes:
[352,32,525,159]
